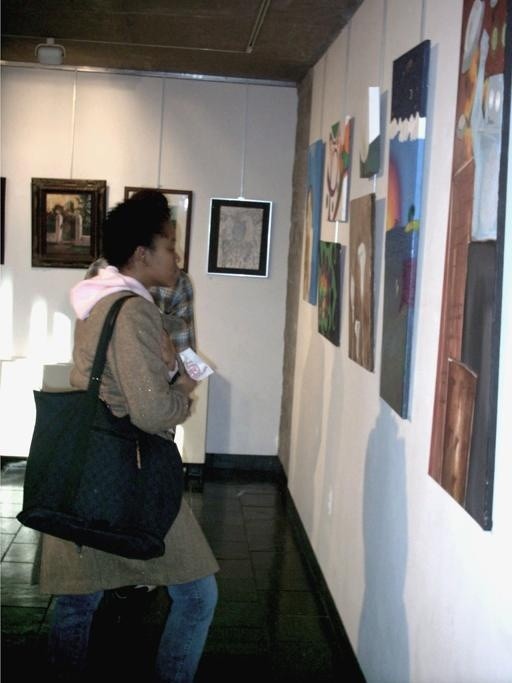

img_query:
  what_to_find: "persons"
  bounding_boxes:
[84,188,195,612]
[53,200,83,243]
[33,194,223,683]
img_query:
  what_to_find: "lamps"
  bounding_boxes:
[32,39,67,65]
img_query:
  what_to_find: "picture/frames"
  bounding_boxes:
[122,185,194,275]
[207,195,272,280]
[29,176,108,269]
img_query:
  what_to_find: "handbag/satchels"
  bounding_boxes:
[16,387,185,560]
